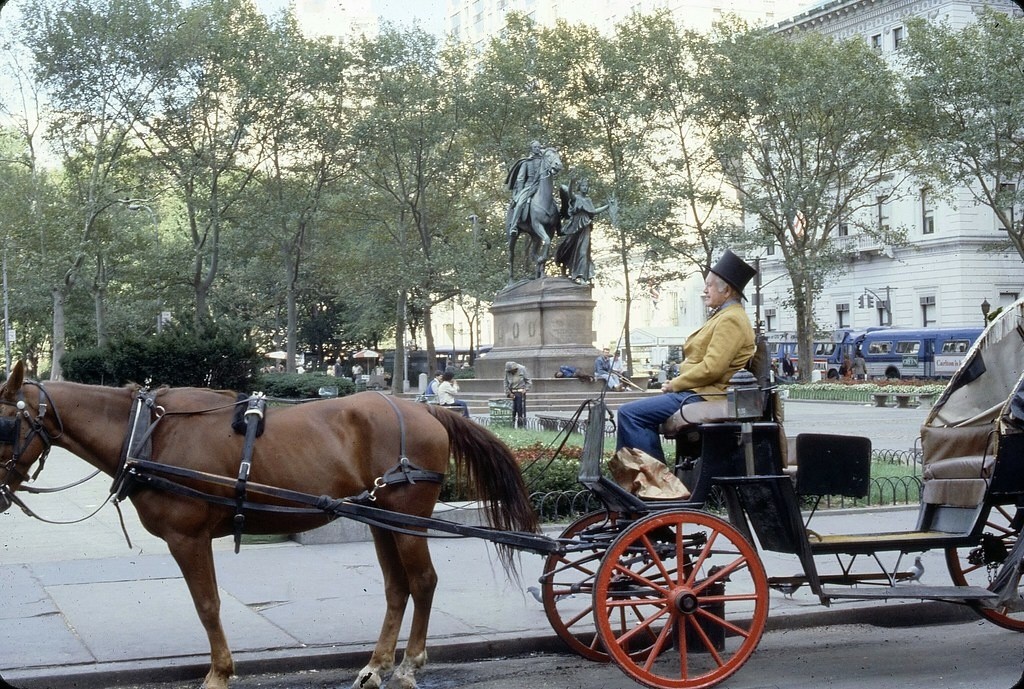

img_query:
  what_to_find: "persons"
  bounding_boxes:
[594,348,625,392]
[334,359,343,377]
[852,352,867,380]
[503,361,532,429]
[278,363,284,373]
[352,362,363,378]
[439,372,470,418]
[783,354,794,377]
[555,177,610,285]
[510,140,566,237]
[270,365,275,374]
[610,350,632,391]
[842,355,852,377]
[427,370,443,394]
[771,358,778,374]
[615,247,758,465]
[663,360,677,373]
[555,365,598,383]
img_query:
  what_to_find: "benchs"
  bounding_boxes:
[871,392,936,410]
[442,405,466,417]
[783,433,871,526]
[920,424,998,507]
[536,414,588,434]
[659,334,778,439]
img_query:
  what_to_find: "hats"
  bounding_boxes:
[699,248,759,304]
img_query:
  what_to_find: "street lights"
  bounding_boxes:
[981,297,991,329]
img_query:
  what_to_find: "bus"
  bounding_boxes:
[851,326,984,383]
[761,327,856,383]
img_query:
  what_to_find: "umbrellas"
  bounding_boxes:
[352,349,382,375]
[266,351,287,360]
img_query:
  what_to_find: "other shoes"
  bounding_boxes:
[615,384,633,392]
[589,376,598,383]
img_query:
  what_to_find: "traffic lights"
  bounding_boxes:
[858,294,864,309]
[867,295,875,308]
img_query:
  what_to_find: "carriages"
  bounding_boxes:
[1,280,1024,689]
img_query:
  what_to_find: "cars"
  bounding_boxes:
[622,360,681,390]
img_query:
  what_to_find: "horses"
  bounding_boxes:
[0,357,540,689]
[506,149,562,283]
